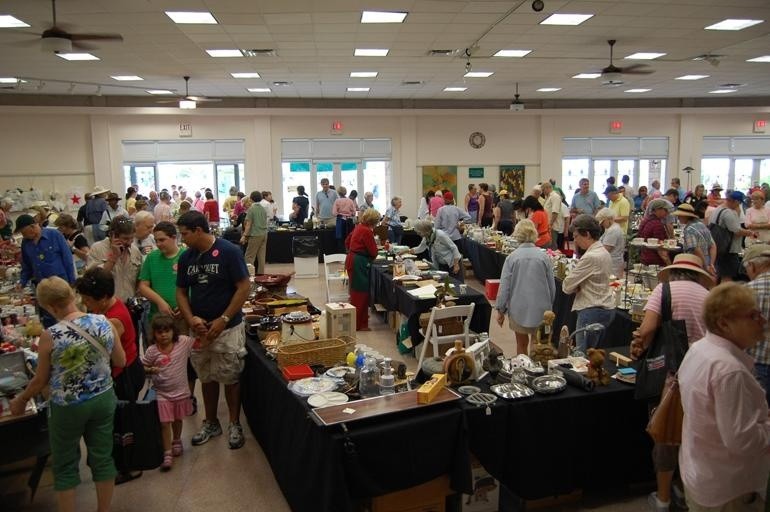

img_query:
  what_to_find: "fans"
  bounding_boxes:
[156,74,224,105]
[481,82,538,113]
[570,39,657,89]
[0,0,124,60]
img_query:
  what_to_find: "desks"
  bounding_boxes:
[0,238,50,451]
[221,209,684,512]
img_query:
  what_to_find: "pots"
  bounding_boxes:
[257,314,282,344]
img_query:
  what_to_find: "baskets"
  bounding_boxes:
[269,339,345,367]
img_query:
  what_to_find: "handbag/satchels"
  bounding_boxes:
[646,372,681,444]
[634,321,688,399]
[106,220,110,237]
[72,253,87,273]
[708,222,734,262]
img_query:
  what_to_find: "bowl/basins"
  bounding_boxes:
[253,274,291,288]
[255,298,277,308]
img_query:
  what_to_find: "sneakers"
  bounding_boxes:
[191,417,245,449]
[648,486,688,512]
[187,395,197,415]
[160,440,183,470]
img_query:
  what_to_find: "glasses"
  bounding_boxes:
[173,194,179,196]
[571,231,587,239]
[83,272,106,296]
[324,192,328,198]
[721,309,762,319]
[112,218,132,227]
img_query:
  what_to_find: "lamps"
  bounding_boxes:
[600,72,623,88]
[13,76,103,98]
[39,36,73,56]
[178,99,197,109]
[462,44,479,75]
[510,103,524,112]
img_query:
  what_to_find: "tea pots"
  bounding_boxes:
[5,264,21,280]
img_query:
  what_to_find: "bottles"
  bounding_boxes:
[384,241,389,252]
[379,356,396,396]
[359,359,380,398]
[355,352,366,378]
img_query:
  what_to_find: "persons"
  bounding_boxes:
[741,242,770,404]
[677,284,770,511]
[1,177,279,512]
[315,175,770,356]
[629,252,715,511]
[290,186,309,225]
[483,348,503,372]
[452,358,470,380]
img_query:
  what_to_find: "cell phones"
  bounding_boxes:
[115,234,123,252]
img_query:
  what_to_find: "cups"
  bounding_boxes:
[0,279,41,336]
[647,238,658,246]
[665,240,677,248]
[634,264,642,271]
[460,284,467,295]
[354,343,385,368]
[389,265,394,274]
[634,237,644,244]
[496,241,503,251]
[433,276,442,283]
[649,265,657,273]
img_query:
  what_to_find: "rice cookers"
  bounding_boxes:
[281,311,315,346]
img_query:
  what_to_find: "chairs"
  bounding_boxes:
[323,252,356,307]
[412,302,478,376]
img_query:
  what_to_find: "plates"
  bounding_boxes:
[646,271,659,277]
[663,245,681,250]
[327,366,358,378]
[645,245,662,249]
[631,270,645,275]
[532,374,567,393]
[294,377,336,393]
[631,243,646,246]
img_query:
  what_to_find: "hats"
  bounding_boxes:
[601,185,617,194]
[730,190,750,206]
[237,191,245,196]
[31,201,48,207]
[14,214,35,231]
[669,203,699,219]
[180,188,187,193]
[710,184,723,191]
[648,199,673,209]
[497,189,508,197]
[105,192,122,201]
[409,218,432,233]
[657,253,714,287]
[738,243,770,274]
[89,185,110,198]
[444,191,453,200]
